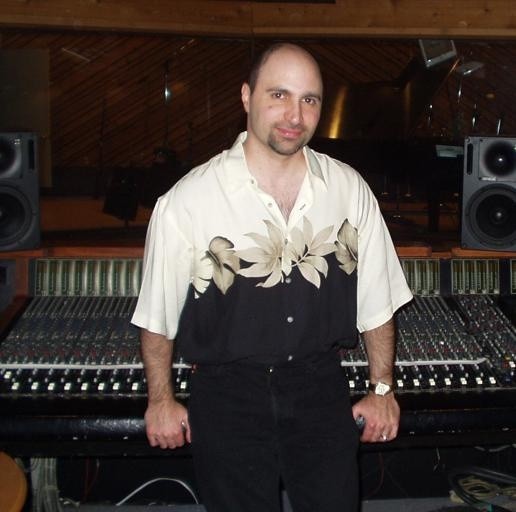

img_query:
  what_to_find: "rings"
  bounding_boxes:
[380,434,388,441]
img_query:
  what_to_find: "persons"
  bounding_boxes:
[130,44,413,512]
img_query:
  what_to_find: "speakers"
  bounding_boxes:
[463,136,516,252]
[0,132,44,252]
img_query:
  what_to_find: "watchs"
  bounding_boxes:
[369,382,394,396]
